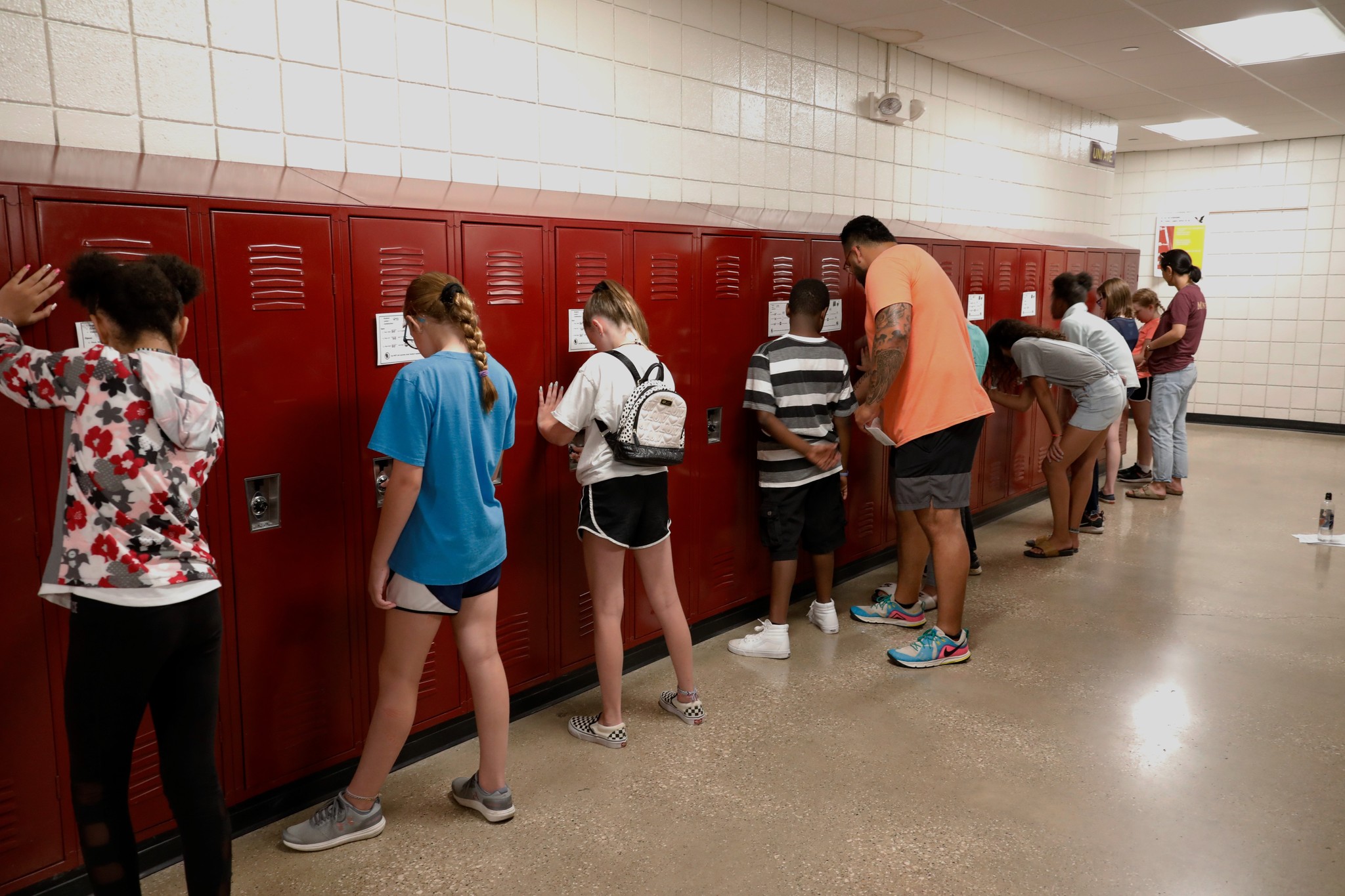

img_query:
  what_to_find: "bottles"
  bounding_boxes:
[1318,492,1335,543]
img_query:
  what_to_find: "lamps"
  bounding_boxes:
[870,92,926,126]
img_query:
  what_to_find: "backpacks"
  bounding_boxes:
[594,350,688,467]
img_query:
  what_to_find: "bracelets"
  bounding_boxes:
[1146,346,1154,351]
[1142,357,1148,362]
[839,470,849,476]
[1051,434,1063,437]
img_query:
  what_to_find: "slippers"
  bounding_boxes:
[918,591,938,611]
[1023,540,1074,558]
[1026,534,1078,552]
[871,582,922,603]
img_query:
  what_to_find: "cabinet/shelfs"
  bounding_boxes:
[0,141,1142,896]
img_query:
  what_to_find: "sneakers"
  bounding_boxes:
[1079,516,1105,534]
[727,618,791,660]
[806,598,839,634]
[451,769,515,822]
[281,790,386,851]
[1089,510,1106,526]
[887,625,971,668]
[850,593,927,627]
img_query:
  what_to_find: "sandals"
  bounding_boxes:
[1165,485,1183,495]
[1125,484,1167,500]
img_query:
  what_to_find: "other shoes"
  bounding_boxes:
[568,712,628,749]
[1117,462,1141,475]
[1097,491,1116,504]
[1117,468,1153,483]
[969,556,982,575]
[659,690,705,725]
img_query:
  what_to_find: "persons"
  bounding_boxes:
[1116,288,1165,483]
[277,272,523,854]
[1096,277,1139,354]
[1079,457,1106,533]
[837,216,990,670]
[535,282,706,750]
[0,250,227,896]
[1124,249,1207,500]
[1047,271,1134,504]
[726,278,856,658]
[982,318,1127,557]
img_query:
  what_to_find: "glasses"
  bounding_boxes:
[1096,298,1102,307]
[843,245,860,274]
